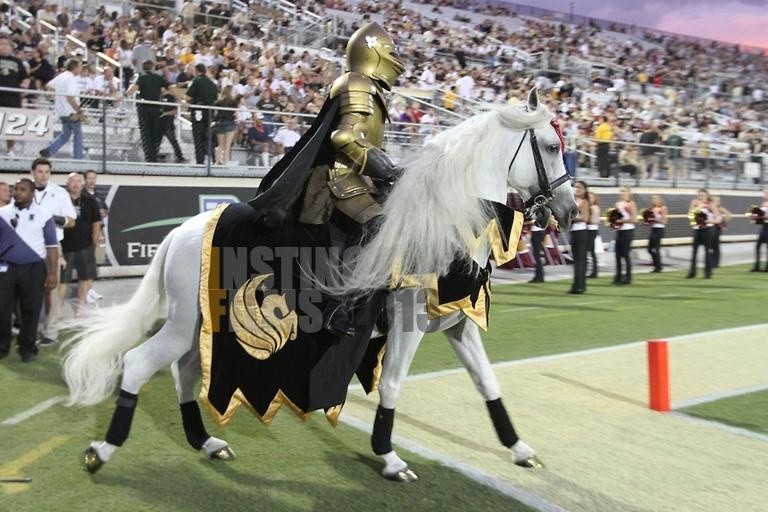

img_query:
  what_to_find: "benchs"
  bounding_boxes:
[0,1,766,186]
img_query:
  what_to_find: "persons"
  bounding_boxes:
[605,185,638,285]
[710,196,732,267]
[566,180,590,293]
[684,188,723,278]
[588,191,601,278]
[248,21,406,335]
[744,187,767,272]
[523,208,560,282]
[2,157,111,362]
[637,193,668,273]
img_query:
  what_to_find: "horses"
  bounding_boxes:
[47,86,581,483]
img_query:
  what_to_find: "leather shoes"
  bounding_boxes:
[323,296,357,337]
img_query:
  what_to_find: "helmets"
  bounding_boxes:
[345,21,407,92]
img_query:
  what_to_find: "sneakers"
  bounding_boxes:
[85,288,102,303]
